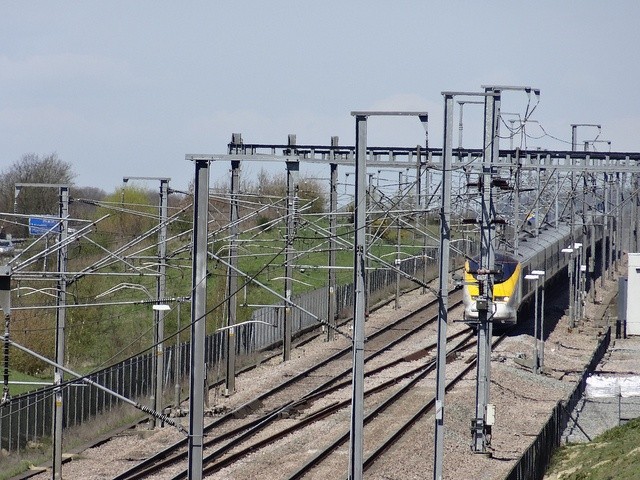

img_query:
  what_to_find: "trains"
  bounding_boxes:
[461,208,615,331]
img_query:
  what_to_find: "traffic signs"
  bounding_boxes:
[29,215,61,234]
